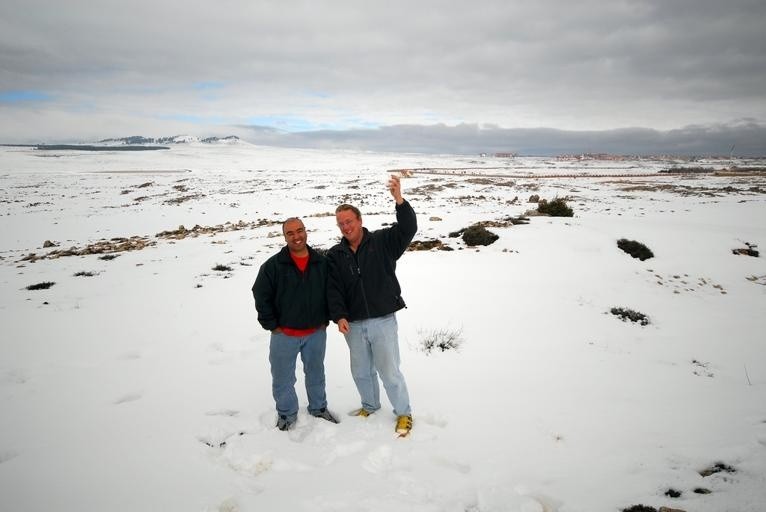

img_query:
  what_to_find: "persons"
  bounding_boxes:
[252,219,339,429]
[324,175,417,436]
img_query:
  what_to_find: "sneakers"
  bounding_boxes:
[396,415,413,436]
[355,408,370,418]
[310,409,337,425]
[276,412,296,431]
